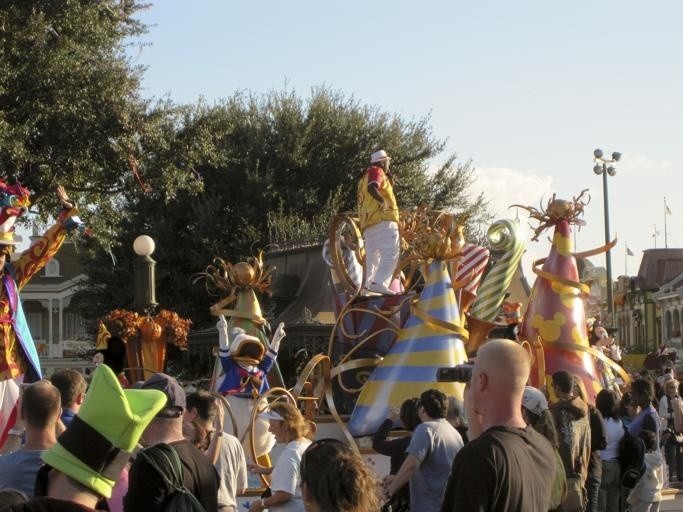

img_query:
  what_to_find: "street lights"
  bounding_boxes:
[593,148,622,328]
[129,234,158,314]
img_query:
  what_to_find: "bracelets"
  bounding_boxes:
[7,429,24,437]
[261,498,269,508]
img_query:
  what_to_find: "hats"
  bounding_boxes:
[370,149,392,164]
[39,363,168,499]
[0,225,23,245]
[521,386,550,417]
[257,409,285,421]
[622,392,632,405]
[130,372,186,418]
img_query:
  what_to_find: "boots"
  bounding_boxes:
[668,464,674,482]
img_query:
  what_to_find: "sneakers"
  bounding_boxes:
[369,283,396,295]
[362,289,384,297]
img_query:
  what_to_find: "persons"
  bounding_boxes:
[245,402,312,512]
[520,359,683,512]
[0,184,78,450]
[357,149,402,296]
[440,340,557,512]
[0,381,64,496]
[183,392,248,511]
[298,438,380,512]
[443,395,469,447]
[372,397,422,476]
[123,372,221,512]
[50,368,88,426]
[5,363,169,511]
[388,389,463,510]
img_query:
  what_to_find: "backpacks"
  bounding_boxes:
[620,416,647,489]
[136,442,206,512]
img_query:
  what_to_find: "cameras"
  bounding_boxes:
[437,363,472,383]
[488,322,520,340]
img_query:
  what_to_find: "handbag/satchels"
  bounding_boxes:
[659,429,672,445]
[671,432,683,445]
[558,472,587,512]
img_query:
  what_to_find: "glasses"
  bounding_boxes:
[300,438,347,487]
[551,382,556,386]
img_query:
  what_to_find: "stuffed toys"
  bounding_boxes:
[90,337,131,389]
[588,320,623,368]
[217,314,288,442]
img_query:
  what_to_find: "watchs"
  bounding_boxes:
[383,487,391,499]
